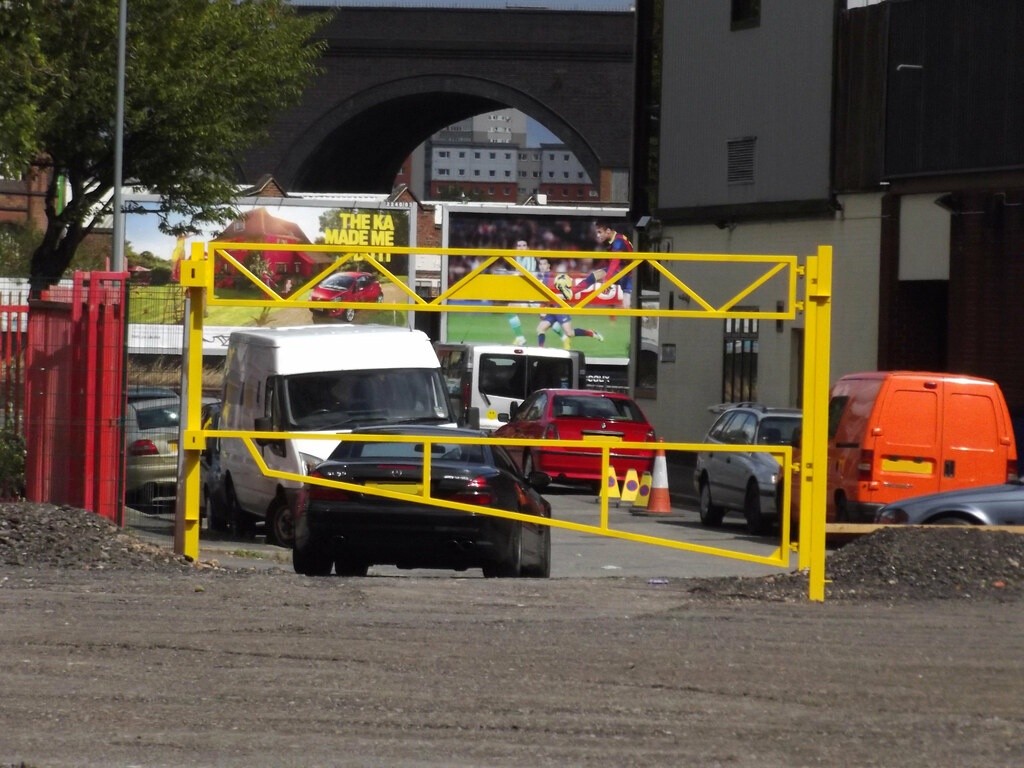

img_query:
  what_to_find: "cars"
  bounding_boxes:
[291,421,552,581]
[125,381,223,540]
[874,471,1024,528]
[493,387,657,494]
[306,269,382,320]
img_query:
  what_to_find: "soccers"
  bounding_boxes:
[553,273,572,291]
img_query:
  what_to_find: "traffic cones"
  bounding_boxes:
[595,465,620,503]
[616,468,640,507]
[628,439,684,519]
[628,471,652,512]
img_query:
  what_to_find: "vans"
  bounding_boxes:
[774,370,1017,546]
[216,323,480,549]
[434,342,578,436]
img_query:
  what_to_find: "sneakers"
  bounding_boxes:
[513,336,526,346]
[590,328,603,342]
[561,334,571,351]
[558,283,573,300]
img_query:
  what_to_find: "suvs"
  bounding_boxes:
[692,403,803,536]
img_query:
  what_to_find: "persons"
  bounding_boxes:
[508,218,633,347]
